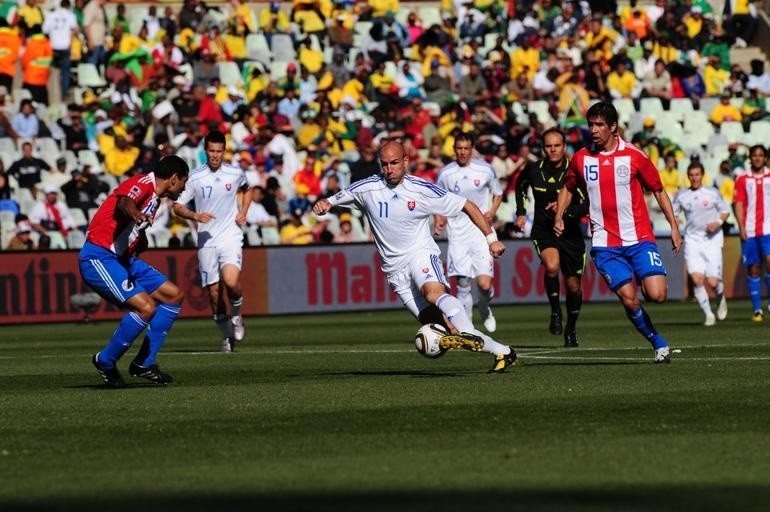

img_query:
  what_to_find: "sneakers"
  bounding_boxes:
[565,328,578,346]
[753,311,763,322]
[655,345,670,363]
[93,352,125,388]
[129,360,173,384]
[550,308,562,335]
[231,315,245,341]
[222,337,233,353]
[439,306,516,374]
[717,299,728,320]
[704,312,715,327]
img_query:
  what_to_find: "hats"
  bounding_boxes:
[644,118,654,127]
[720,90,731,96]
[44,186,59,193]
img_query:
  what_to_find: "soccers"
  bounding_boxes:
[413,322,447,359]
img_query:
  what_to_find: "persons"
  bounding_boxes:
[553,99,682,362]
[313,140,517,375]
[0,1,769,252]
[514,128,590,348]
[673,164,731,326]
[79,156,188,386]
[435,134,503,333]
[173,130,254,352]
[735,144,770,322]
